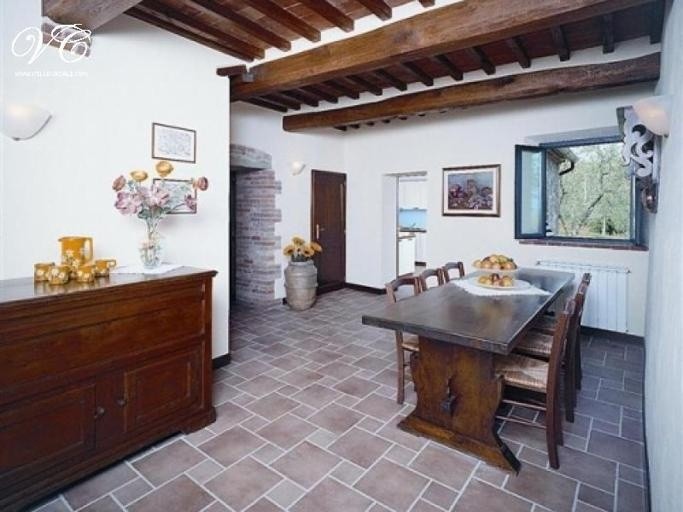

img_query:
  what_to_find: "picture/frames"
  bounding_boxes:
[149,122,197,165]
[440,162,501,218]
[152,177,198,216]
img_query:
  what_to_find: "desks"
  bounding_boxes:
[360,265,575,474]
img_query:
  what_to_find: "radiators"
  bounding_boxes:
[536,258,634,336]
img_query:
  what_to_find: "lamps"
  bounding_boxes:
[0,101,53,143]
[631,92,672,141]
[290,161,306,175]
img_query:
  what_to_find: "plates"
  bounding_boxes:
[472,266,519,273]
[475,281,516,289]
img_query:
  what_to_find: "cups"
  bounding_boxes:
[33,259,115,286]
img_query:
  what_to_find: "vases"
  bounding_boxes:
[136,234,165,268]
[283,260,319,311]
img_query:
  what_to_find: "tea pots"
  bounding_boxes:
[57,237,94,279]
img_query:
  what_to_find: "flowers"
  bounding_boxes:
[110,159,210,260]
[281,236,322,263]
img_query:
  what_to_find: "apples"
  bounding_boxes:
[475,255,515,286]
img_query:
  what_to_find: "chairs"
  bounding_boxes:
[496,294,578,473]
[417,265,443,292]
[439,260,467,285]
[536,273,593,394]
[518,284,582,421]
[384,273,422,405]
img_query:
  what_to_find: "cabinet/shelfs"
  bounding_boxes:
[0,256,218,512]
[397,233,416,277]
